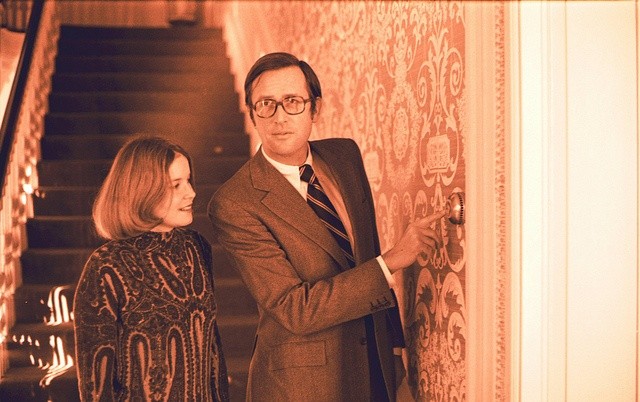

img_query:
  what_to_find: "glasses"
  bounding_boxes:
[251,95,312,118]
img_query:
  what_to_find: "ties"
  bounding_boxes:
[299,164,355,268]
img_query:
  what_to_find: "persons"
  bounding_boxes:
[207,52,446,401]
[73,134,230,402]
